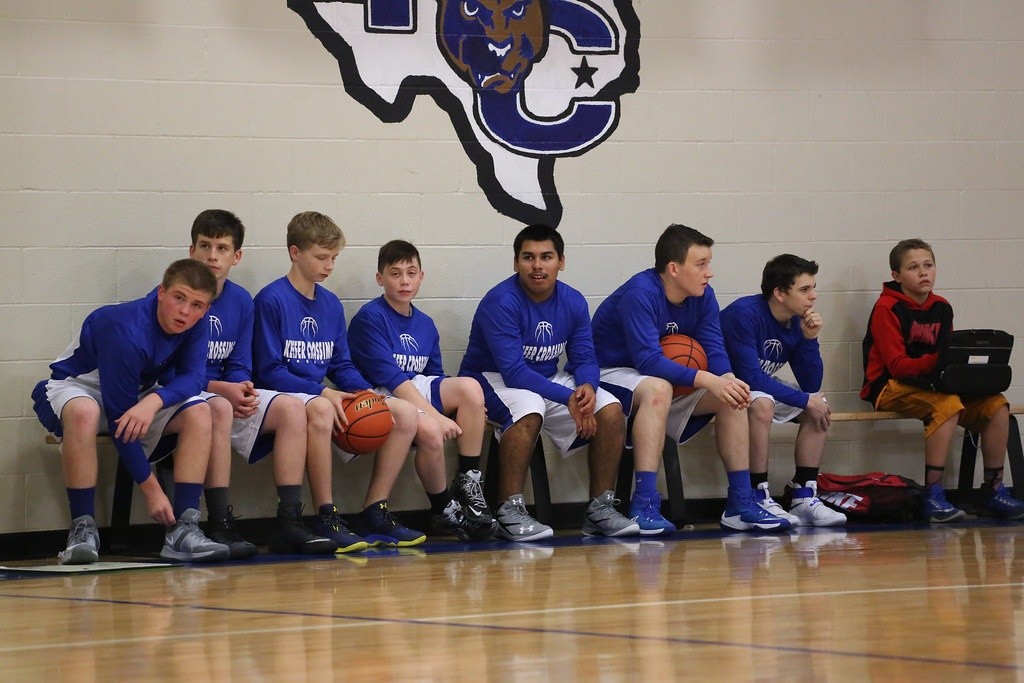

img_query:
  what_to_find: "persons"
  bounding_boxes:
[457,224,639,541]
[146,209,339,555]
[252,211,426,551]
[347,239,500,540]
[31,259,232,564]
[562,224,791,533]
[720,253,847,527]
[860,239,1024,522]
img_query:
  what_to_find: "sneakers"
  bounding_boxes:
[788,478,847,526]
[719,494,790,532]
[208,504,257,559]
[975,482,1024,521]
[312,504,368,552]
[269,503,338,555]
[754,482,798,526]
[917,488,964,524]
[62,514,100,565]
[627,491,674,535]
[355,500,427,548]
[581,490,640,537]
[491,493,554,542]
[161,508,230,563]
[451,469,493,524]
[430,497,498,542]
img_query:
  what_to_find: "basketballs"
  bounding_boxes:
[332,390,392,455]
[660,333,708,394]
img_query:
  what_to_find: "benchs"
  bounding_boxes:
[43,404,1024,558]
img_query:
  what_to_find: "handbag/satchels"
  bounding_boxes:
[817,471,924,524]
[901,329,1014,393]
